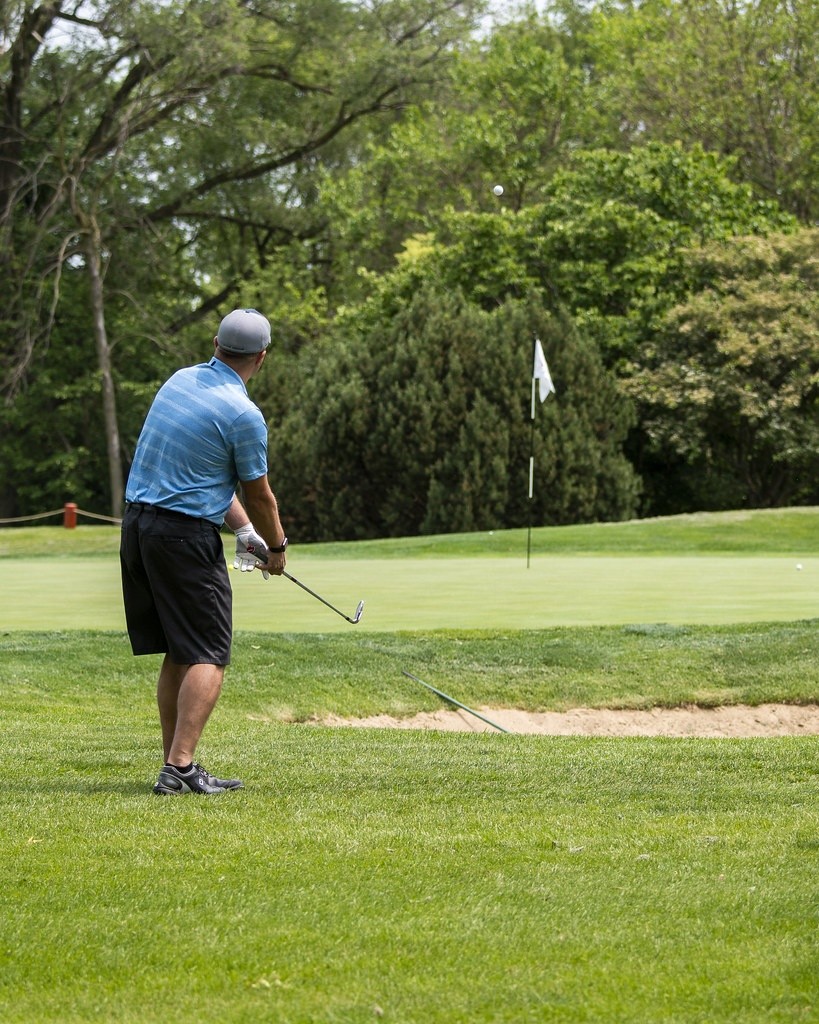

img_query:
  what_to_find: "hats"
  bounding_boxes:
[217,308,271,355]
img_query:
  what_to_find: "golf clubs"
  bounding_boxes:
[246,543,365,625]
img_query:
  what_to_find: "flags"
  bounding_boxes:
[533,340,555,403]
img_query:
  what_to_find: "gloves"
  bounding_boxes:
[233,522,269,580]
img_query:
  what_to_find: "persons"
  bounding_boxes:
[119,308,286,796]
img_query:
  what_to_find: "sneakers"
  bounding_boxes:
[194,764,242,790]
[153,766,226,795]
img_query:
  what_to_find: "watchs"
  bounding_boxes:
[269,537,288,553]
